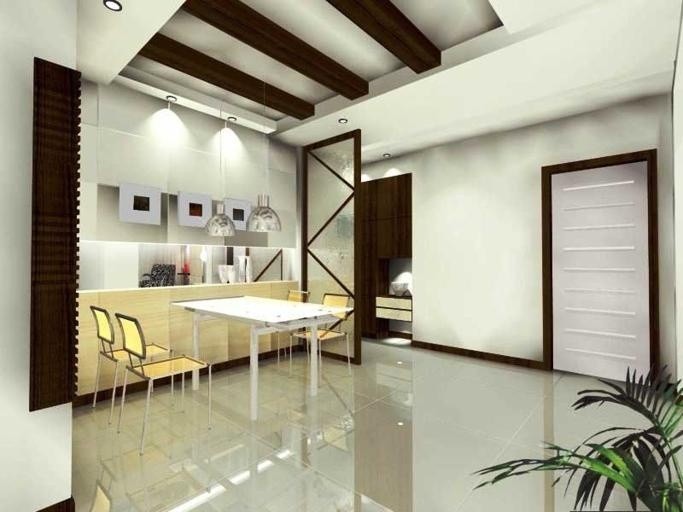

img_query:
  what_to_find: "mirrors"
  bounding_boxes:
[138,244,283,288]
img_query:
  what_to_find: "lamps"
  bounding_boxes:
[206,99,237,237]
[246,72,282,232]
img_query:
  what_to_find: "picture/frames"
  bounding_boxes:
[119,182,161,226]
[177,191,213,228]
[222,198,252,230]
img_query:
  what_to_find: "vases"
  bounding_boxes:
[238,255,246,283]
[219,264,229,284]
[228,265,239,283]
[245,255,254,283]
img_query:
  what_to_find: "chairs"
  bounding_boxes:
[88,455,129,512]
[139,263,176,287]
[117,312,212,456]
[90,307,174,424]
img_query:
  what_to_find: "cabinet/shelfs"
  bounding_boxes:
[375,296,412,322]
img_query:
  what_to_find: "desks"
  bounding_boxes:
[168,293,354,422]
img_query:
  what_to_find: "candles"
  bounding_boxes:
[201,248,208,285]
[184,245,189,273]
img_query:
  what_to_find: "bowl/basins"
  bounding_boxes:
[391,282,409,296]
